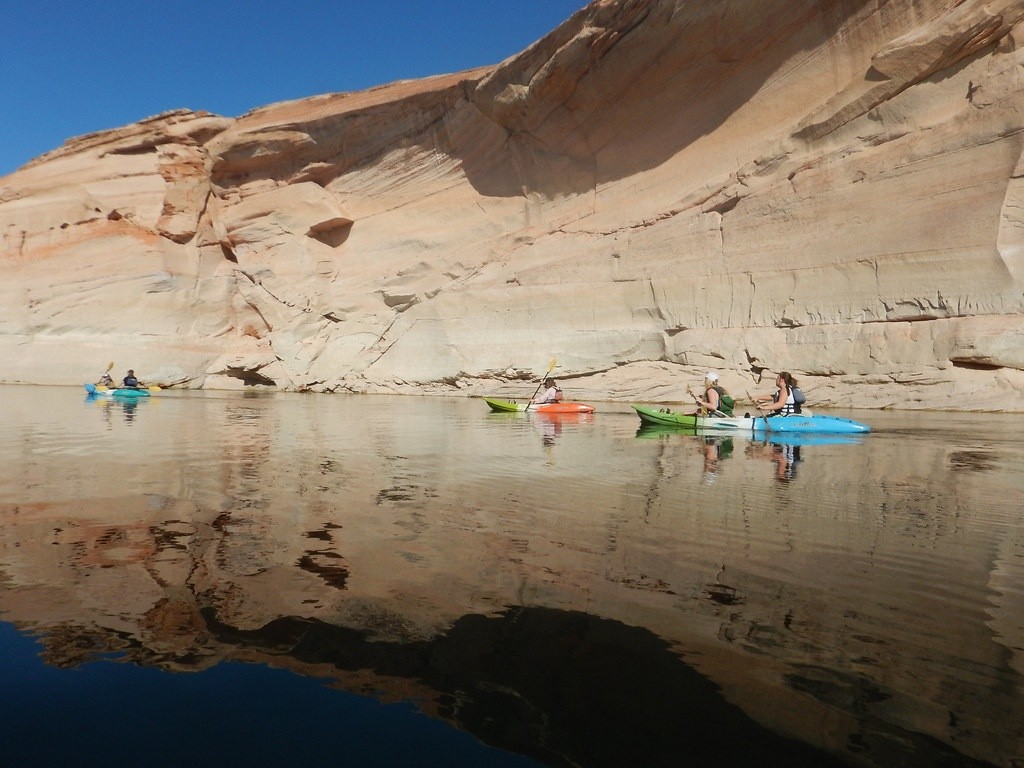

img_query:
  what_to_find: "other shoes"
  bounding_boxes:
[666,408,672,414]
[660,409,665,412]
[744,412,750,418]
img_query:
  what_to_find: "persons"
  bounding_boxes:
[744,372,802,418]
[118,369,139,389]
[508,377,559,407]
[660,371,730,418]
[95,374,116,386]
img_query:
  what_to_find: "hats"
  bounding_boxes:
[102,374,110,378]
[705,371,719,382]
[127,370,134,373]
[543,377,553,385]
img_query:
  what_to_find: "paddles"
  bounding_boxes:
[685,383,707,417]
[745,390,773,434]
[94,361,115,386]
[524,359,557,411]
[95,379,163,392]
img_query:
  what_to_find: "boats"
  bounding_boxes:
[84,383,151,398]
[631,404,872,434]
[483,397,595,415]
